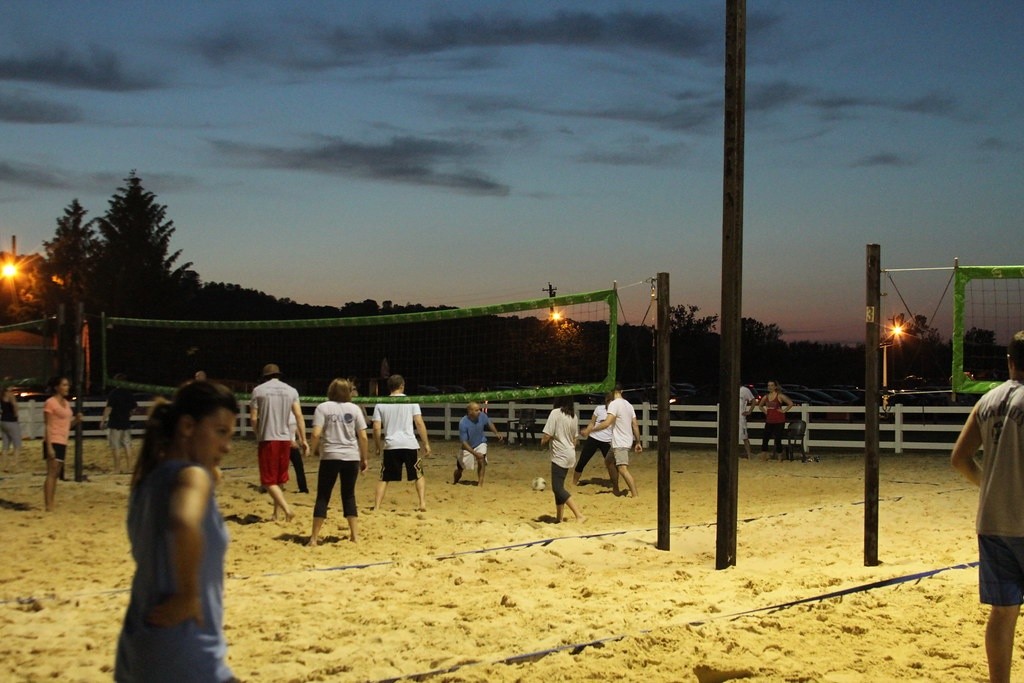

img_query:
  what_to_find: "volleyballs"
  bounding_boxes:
[531,476,546,492]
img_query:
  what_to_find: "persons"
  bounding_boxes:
[951,328,1024,683]
[739,385,755,460]
[0,375,24,474]
[113,378,244,683]
[453,402,503,485]
[590,386,642,497]
[196,372,205,381]
[42,378,83,511]
[305,378,368,547]
[541,395,588,525]
[372,375,431,511]
[573,391,619,487]
[100,381,139,474]
[249,364,310,522]
[759,381,792,453]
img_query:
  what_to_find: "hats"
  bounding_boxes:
[263,364,282,376]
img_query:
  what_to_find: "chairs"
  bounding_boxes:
[782,418,807,462]
[506,408,537,447]
